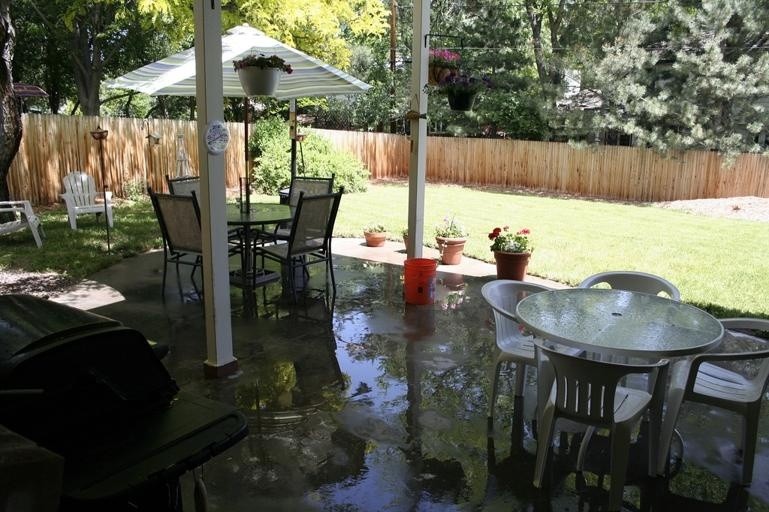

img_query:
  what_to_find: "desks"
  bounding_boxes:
[60,387,249,512]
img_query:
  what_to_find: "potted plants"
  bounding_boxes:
[402,228,409,250]
[364,224,387,246]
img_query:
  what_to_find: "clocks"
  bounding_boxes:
[204,120,231,154]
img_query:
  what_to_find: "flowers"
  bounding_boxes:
[429,48,461,62]
[435,216,470,238]
[488,225,535,253]
[233,54,293,74]
[437,73,495,96]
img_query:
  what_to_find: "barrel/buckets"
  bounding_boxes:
[402,302,436,341]
[403,257,440,305]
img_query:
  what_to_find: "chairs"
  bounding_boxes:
[58,171,113,230]
[147,173,345,318]
[0,200,47,249]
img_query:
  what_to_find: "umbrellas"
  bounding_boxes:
[101,20,369,212]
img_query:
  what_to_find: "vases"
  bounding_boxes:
[447,89,478,111]
[429,63,457,85]
[494,251,531,280]
[436,236,467,265]
